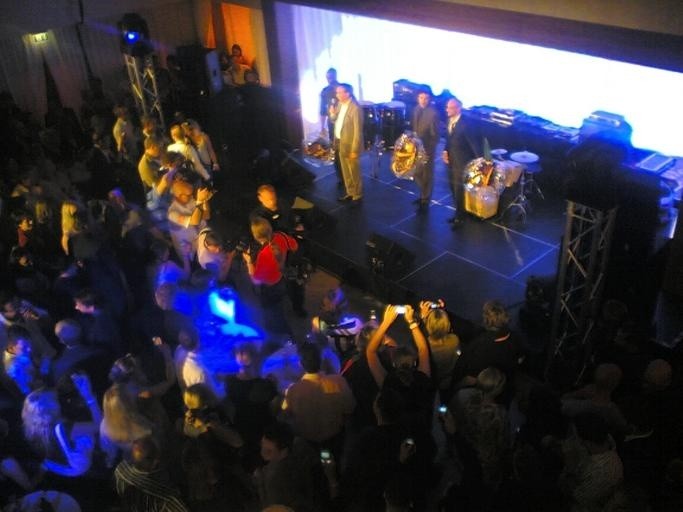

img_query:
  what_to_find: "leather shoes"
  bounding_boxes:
[338,195,352,201]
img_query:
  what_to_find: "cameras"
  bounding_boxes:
[236,241,248,252]
[396,304,408,314]
[430,302,441,309]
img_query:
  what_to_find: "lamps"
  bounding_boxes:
[290,194,337,235]
[366,233,415,275]
[569,110,636,196]
[112,15,155,62]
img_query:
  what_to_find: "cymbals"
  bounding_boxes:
[510,152,538,163]
[490,149,507,155]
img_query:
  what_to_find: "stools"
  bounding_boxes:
[523,165,546,201]
[489,147,508,220]
[502,150,542,227]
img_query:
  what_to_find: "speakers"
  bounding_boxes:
[159,45,257,181]
[278,150,316,186]
[519,175,669,338]
[313,205,336,234]
[366,235,406,271]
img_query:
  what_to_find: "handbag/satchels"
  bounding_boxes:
[280,231,312,288]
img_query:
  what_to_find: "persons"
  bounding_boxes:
[412,88,440,212]
[441,97,483,226]
[331,83,363,204]
[283,287,683,512]
[225,44,260,88]
[319,67,346,189]
[1,80,344,510]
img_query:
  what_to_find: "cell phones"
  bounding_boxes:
[320,450,331,466]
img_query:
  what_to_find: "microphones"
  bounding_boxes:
[16,304,40,321]
[331,97,336,107]
